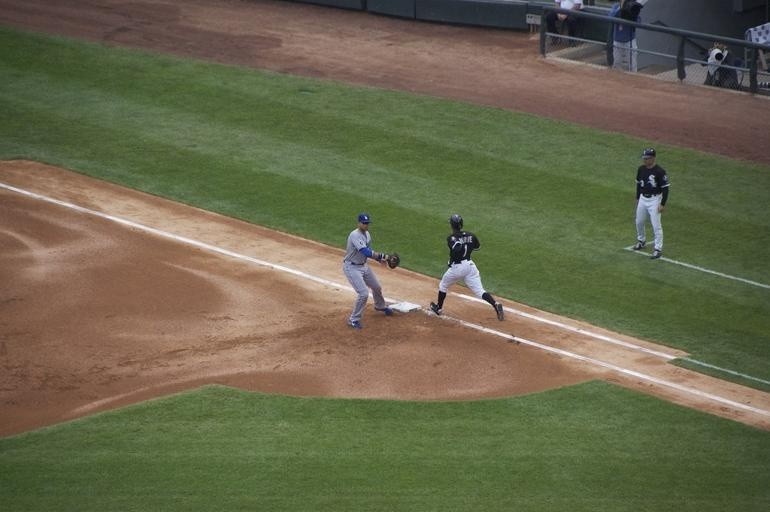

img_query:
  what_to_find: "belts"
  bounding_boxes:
[455,258,470,264]
[344,260,366,265]
[643,193,656,197]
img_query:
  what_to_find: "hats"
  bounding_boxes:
[641,147,656,159]
[359,214,372,223]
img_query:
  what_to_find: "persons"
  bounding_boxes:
[701,42,745,90]
[431,214,504,322]
[341,213,393,328]
[632,148,669,259]
[609,0,642,72]
[546,0,586,48]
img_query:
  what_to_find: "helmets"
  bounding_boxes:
[450,215,463,228]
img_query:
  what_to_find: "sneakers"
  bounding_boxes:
[634,240,646,250]
[650,249,662,259]
[348,318,361,328]
[494,301,504,321]
[430,302,442,315]
[374,306,393,316]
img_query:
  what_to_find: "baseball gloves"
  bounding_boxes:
[387,252,399,268]
